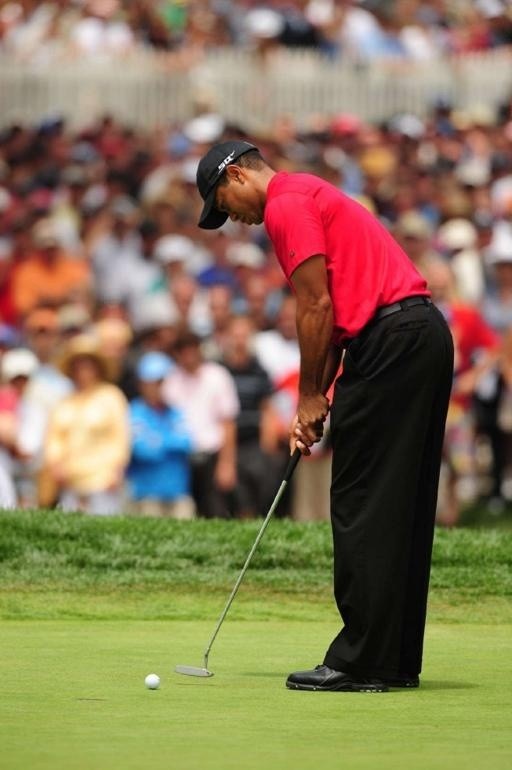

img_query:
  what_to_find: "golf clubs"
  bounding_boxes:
[175,447,301,678]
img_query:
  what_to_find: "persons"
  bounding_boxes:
[197,140,454,692]
[1,1,510,526]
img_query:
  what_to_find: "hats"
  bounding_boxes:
[193,138,261,231]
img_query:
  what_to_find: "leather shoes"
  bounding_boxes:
[285,662,421,693]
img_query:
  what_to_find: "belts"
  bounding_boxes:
[373,294,433,325]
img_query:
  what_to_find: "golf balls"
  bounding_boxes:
[145,673,159,687]
